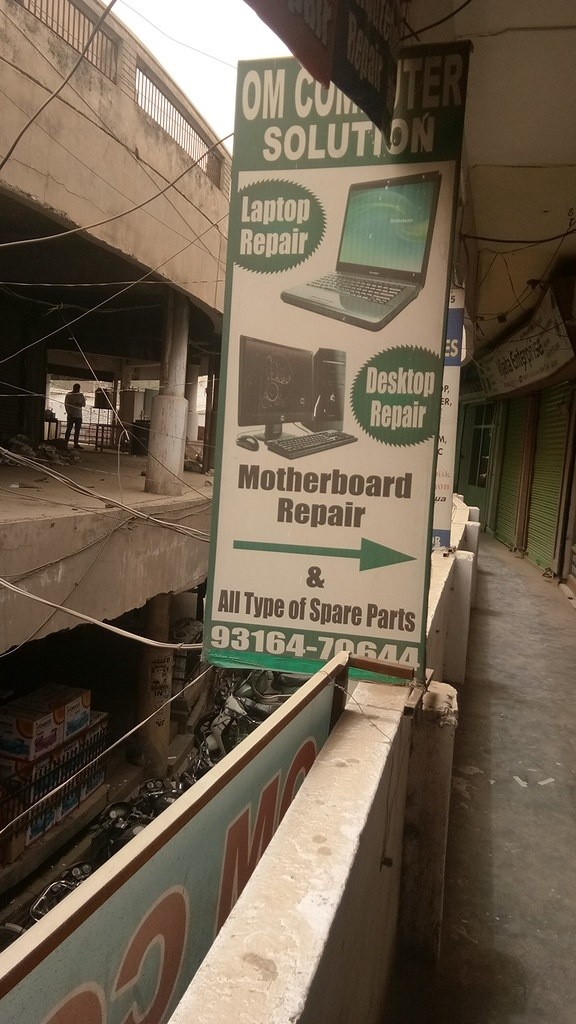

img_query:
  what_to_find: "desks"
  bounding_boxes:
[95,424,132,455]
[45,420,58,448]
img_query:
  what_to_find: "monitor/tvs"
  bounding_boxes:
[237,335,315,442]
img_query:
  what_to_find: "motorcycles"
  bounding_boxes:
[0,661,317,970]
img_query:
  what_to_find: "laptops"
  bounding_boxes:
[280,170,442,332]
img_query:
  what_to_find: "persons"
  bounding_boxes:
[64,382,86,449]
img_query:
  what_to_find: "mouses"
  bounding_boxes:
[236,435,259,451]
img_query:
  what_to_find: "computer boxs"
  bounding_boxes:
[302,348,346,432]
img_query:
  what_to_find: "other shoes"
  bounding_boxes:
[73,444,84,449]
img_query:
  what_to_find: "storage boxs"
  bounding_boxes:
[54,735,86,782]
[56,785,80,823]
[0,799,24,864]
[0,750,62,804]
[80,764,106,803]
[0,697,65,762]
[79,709,113,761]
[26,806,56,847]
[24,682,91,741]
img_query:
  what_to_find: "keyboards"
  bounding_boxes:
[268,432,358,460]
